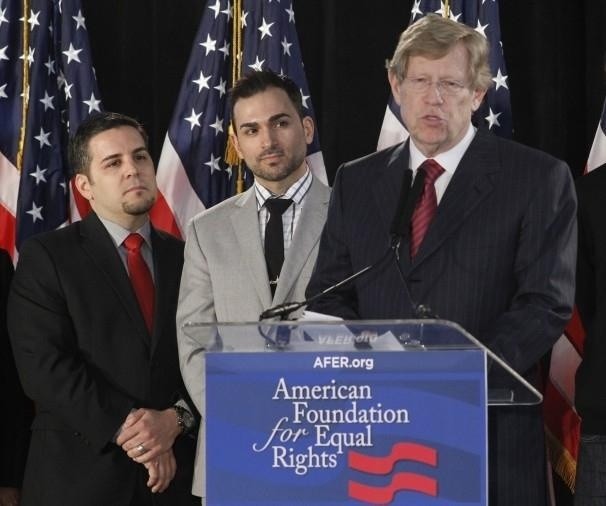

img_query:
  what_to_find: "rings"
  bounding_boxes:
[137,444,145,455]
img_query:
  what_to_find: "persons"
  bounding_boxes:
[173,72,334,505]
[0,247,36,506]
[304,7,579,505]
[7,108,202,506]
[577,103,606,506]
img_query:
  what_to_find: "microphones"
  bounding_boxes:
[392,234,434,345]
[262,236,401,347]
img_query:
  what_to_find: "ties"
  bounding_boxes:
[260,196,293,299]
[406,156,446,264]
[122,233,158,336]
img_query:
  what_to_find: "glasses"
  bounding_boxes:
[397,68,475,93]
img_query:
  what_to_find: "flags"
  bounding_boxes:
[0,0,107,270]
[373,0,587,496]
[149,0,334,242]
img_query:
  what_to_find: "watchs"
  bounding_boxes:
[169,404,194,436]
